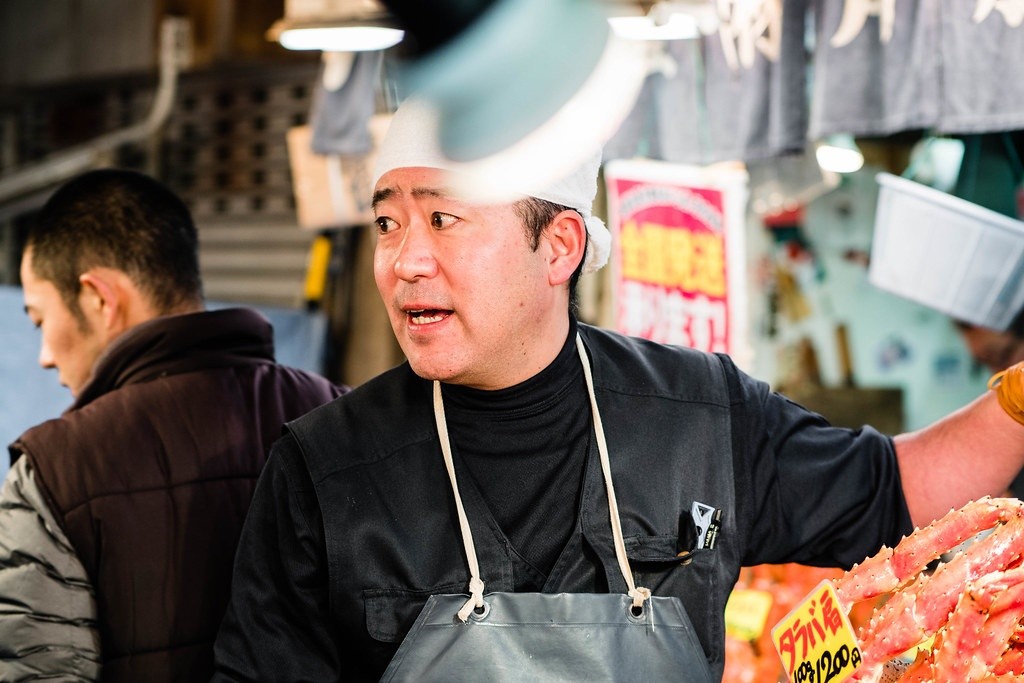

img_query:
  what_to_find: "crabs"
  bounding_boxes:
[816,493,1023,682]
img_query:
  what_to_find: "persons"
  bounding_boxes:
[208,90,1024,683]
[0,168,349,683]
